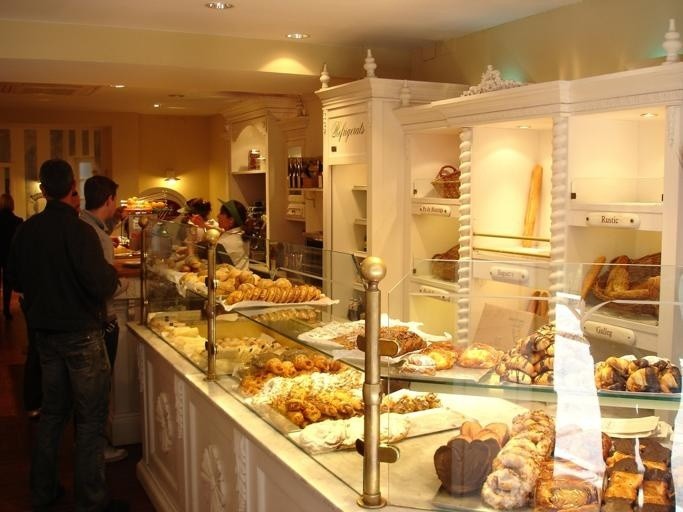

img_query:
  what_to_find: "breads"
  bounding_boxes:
[236,350,440,419]
[435,409,683,511]
[401,341,499,376]
[356,325,426,355]
[594,357,681,393]
[522,165,544,248]
[310,321,364,350]
[154,315,286,359]
[298,411,411,452]
[197,264,292,294]
[495,322,554,385]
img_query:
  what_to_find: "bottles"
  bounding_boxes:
[248,149,266,170]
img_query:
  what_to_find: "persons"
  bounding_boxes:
[14,286,42,417]
[181,198,254,271]
[78,176,126,463]
[72,192,81,214]
[8,158,133,512]
[0,192,24,317]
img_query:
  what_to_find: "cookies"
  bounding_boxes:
[254,308,316,322]
[226,283,321,304]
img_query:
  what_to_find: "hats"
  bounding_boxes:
[219,198,244,227]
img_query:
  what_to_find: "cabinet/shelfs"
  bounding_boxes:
[267,189,323,289]
[408,105,666,366]
[227,117,268,274]
[328,163,370,325]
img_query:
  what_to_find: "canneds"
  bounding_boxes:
[248,147,260,170]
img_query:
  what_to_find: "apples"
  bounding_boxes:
[127,196,165,213]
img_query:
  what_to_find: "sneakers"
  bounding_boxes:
[102,444,129,464]
[33,482,129,512]
